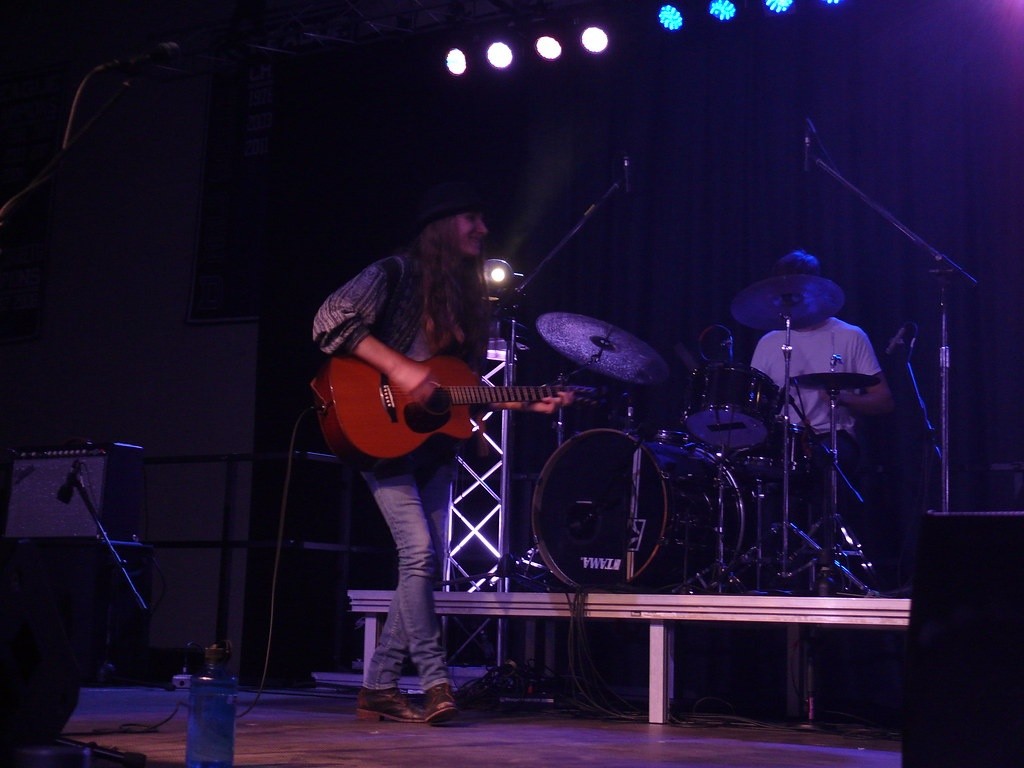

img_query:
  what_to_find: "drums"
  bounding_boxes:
[531,422,748,593]
[733,421,823,485]
[683,361,779,452]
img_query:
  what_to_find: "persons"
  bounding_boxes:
[311,184,575,725]
[751,251,895,580]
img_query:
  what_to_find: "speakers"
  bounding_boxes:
[1,587,83,743]
[903,510,1023,768]
[1,443,144,541]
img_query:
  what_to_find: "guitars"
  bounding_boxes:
[311,349,598,470]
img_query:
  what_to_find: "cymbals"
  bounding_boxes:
[536,311,671,385]
[790,372,881,390]
[728,275,849,331]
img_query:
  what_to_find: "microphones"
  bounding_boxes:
[99,41,179,70]
[623,156,630,193]
[624,399,636,432]
[803,122,811,174]
[773,384,786,415]
[57,462,77,502]
[724,335,734,362]
[885,323,909,355]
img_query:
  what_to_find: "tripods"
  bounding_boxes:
[654,304,886,598]
[438,173,625,594]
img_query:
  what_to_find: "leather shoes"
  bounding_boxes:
[424,684,458,722]
[356,685,426,722]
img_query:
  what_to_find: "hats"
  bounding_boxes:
[413,186,494,225]
